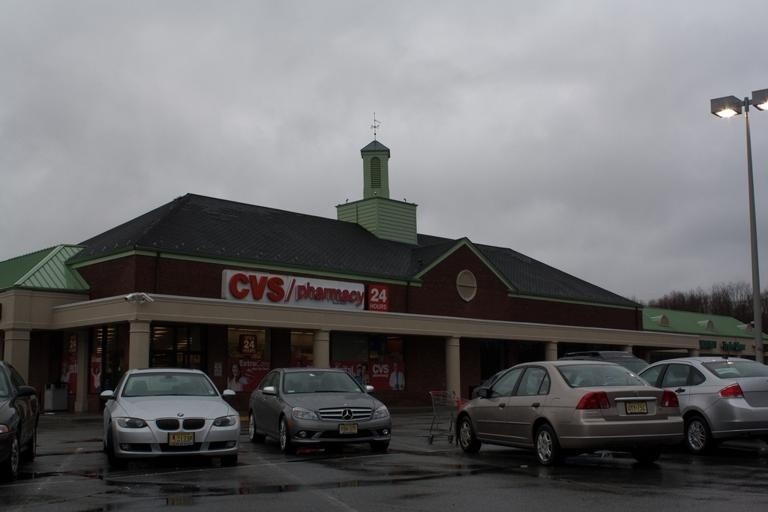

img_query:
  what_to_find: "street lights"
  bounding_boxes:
[709,86,768,371]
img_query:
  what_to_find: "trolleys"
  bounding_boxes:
[426,387,469,447]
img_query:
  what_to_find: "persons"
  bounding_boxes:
[90,364,102,393]
[226,360,250,394]
[388,362,406,391]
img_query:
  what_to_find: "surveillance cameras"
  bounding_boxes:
[141,293,154,304]
[124,291,137,302]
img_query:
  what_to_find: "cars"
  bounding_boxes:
[0,359,43,475]
[246,367,392,454]
[100,368,241,466]
